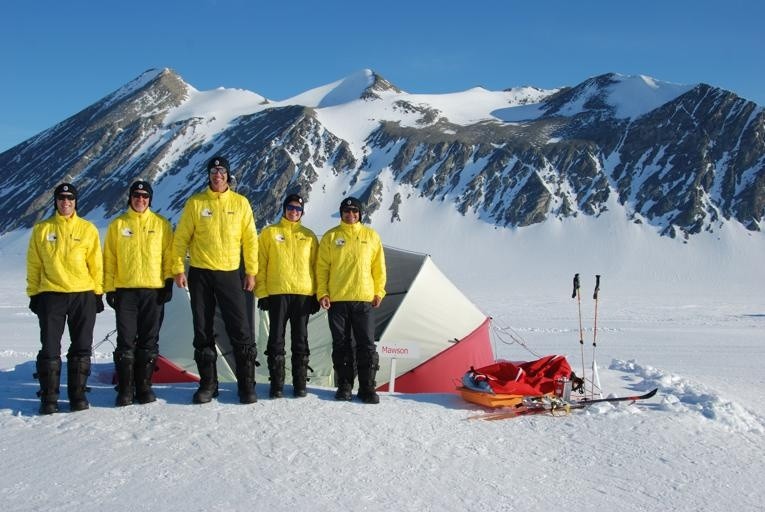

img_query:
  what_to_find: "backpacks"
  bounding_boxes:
[464,354,582,397]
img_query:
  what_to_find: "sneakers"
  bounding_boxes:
[270,386,282,398]
[39,400,59,414]
[135,385,156,403]
[335,384,353,401]
[358,388,379,403]
[69,391,89,412]
[115,387,134,407]
[193,380,219,404]
[238,385,257,404]
[294,384,307,397]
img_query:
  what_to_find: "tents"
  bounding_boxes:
[111,243,493,398]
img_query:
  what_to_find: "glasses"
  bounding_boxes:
[210,167,227,174]
[344,207,359,213]
[287,205,303,211]
[132,192,151,198]
[56,193,75,200]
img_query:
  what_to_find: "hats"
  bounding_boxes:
[54,183,78,194]
[341,197,362,208]
[130,181,153,193]
[208,157,230,168]
[283,194,304,206]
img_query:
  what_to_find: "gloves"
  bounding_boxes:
[257,297,269,311]
[28,295,39,314]
[96,294,103,314]
[157,278,174,305]
[105,291,115,309]
[307,295,320,314]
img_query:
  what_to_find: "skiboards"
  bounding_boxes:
[466,388,659,420]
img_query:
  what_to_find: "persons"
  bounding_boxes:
[102,182,174,407]
[173,156,259,404]
[254,193,322,399]
[314,196,387,404]
[25,183,104,413]
